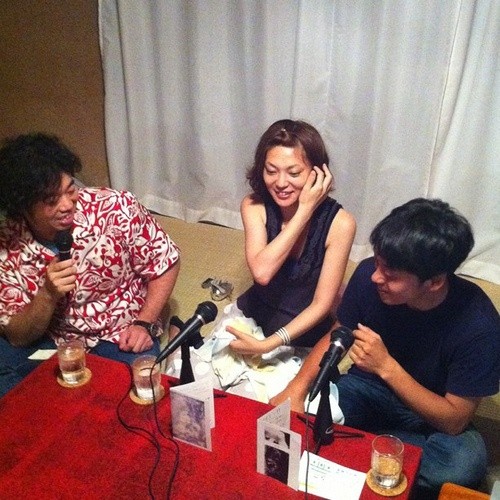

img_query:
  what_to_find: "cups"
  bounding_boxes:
[132,355,162,400]
[371,435,404,488]
[57,340,85,383]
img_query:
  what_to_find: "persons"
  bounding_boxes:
[0,131,181,398]
[268,197,500,500]
[183,119,356,403]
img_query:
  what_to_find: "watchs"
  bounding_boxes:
[132,320,158,341]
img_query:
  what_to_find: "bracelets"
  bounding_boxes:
[275,327,291,346]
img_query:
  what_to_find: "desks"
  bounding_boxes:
[0,349,424,500]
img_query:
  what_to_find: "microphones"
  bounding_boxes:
[155,301,218,364]
[54,231,74,298]
[309,326,356,402]
